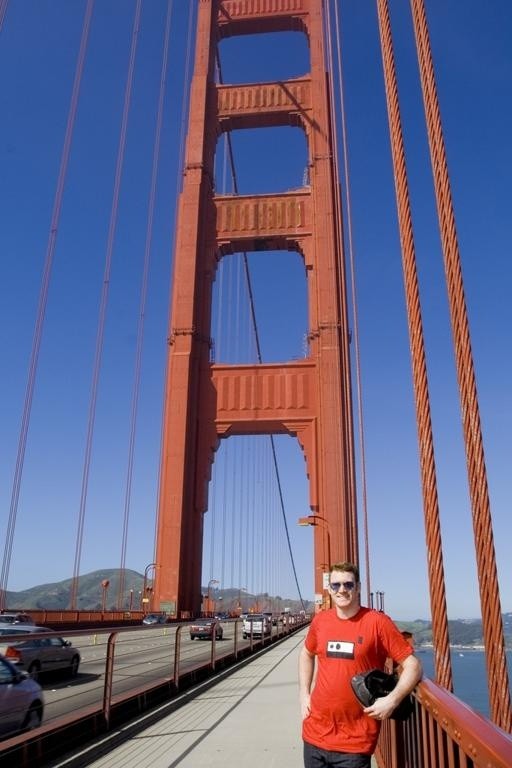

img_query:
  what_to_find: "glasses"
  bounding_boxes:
[328,582,355,591]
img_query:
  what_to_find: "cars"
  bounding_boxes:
[142,614,167,625]
[189,613,228,640]
[0,613,81,742]
[240,612,303,639]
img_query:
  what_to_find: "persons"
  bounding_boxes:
[301,561,422,767]
